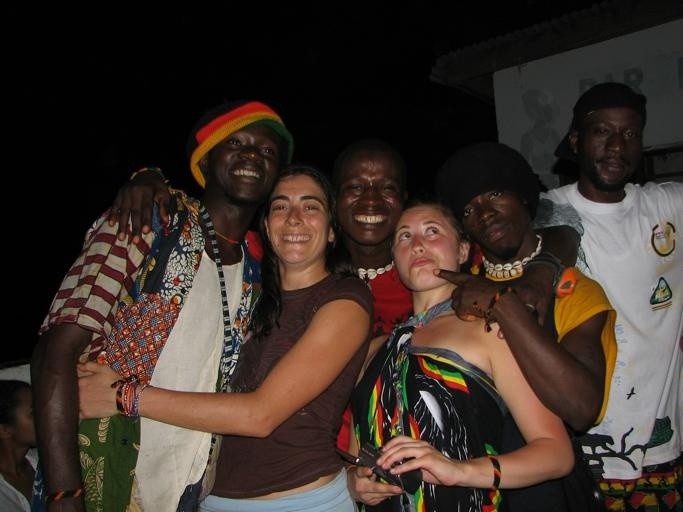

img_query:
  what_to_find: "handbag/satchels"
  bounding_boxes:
[28,410,143,511]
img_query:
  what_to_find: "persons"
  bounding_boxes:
[346,199,575,512]
[30,98,294,512]
[330,140,581,453]
[78,165,376,512]
[531,83,683,512]
[0,380,38,512]
[436,132,617,431]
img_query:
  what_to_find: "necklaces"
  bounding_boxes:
[482,234,542,278]
[358,261,395,279]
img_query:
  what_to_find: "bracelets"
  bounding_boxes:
[129,167,166,182]
[43,488,84,505]
[486,455,500,491]
[346,465,356,472]
[111,375,153,417]
[524,251,564,286]
[474,286,518,333]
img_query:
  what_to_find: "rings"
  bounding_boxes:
[524,303,536,310]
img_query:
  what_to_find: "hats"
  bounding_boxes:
[189,97,294,190]
[431,141,540,224]
[551,79,649,160]
